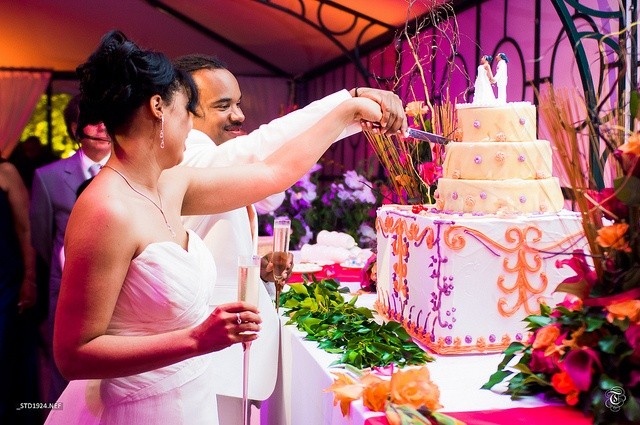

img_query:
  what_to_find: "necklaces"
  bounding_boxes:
[100,165,177,238]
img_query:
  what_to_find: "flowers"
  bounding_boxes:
[322,363,466,425]
[266,164,322,251]
[484,130,640,425]
[385,98,443,205]
[322,169,377,238]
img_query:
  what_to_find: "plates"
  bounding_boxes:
[290,264,323,272]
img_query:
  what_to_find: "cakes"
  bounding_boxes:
[373,53,613,355]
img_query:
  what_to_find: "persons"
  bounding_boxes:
[31,92,113,419]
[0,140,37,371]
[43,27,409,425]
[168,53,409,425]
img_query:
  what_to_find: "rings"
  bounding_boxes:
[235,312,242,325]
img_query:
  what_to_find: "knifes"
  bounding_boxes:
[360,118,455,144]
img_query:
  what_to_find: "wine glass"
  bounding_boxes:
[274,220,291,313]
[237,256,260,425]
